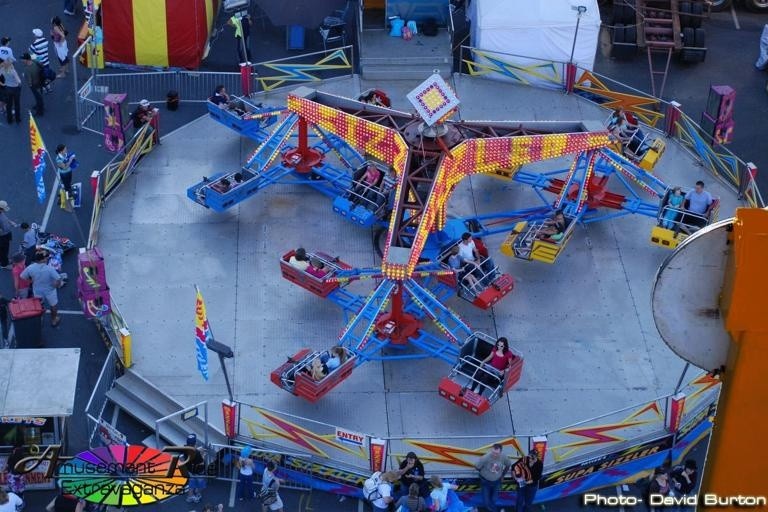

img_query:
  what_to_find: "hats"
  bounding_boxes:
[0,200,11,213]
[0,35,12,46]
[31,28,44,38]
[20,52,32,60]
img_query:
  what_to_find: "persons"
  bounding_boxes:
[606,106,647,156]
[306,256,330,278]
[289,247,311,271]
[470,337,514,395]
[0,430,699,512]
[287,356,329,381]
[363,165,399,211]
[320,345,348,369]
[662,186,684,231]
[364,91,388,108]
[680,180,714,237]
[348,162,380,211]
[448,207,568,297]
[0,0,155,327]
[210,178,231,194]
[231,172,244,188]
[211,8,254,117]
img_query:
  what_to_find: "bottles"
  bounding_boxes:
[414,468,419,481]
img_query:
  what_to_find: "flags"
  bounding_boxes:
[195,293,209,381]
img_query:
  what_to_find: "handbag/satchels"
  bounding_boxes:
[49,30,69,42]
[39,65,57,88]
[513,463,532,489]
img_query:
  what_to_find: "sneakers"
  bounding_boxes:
[51,316,61,328]
[0,258,14,272]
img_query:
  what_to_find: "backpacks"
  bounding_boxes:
[362,474,387,502]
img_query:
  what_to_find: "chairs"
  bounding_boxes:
[314,15,348,59]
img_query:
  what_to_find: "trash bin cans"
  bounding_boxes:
[318,16,353,66]
